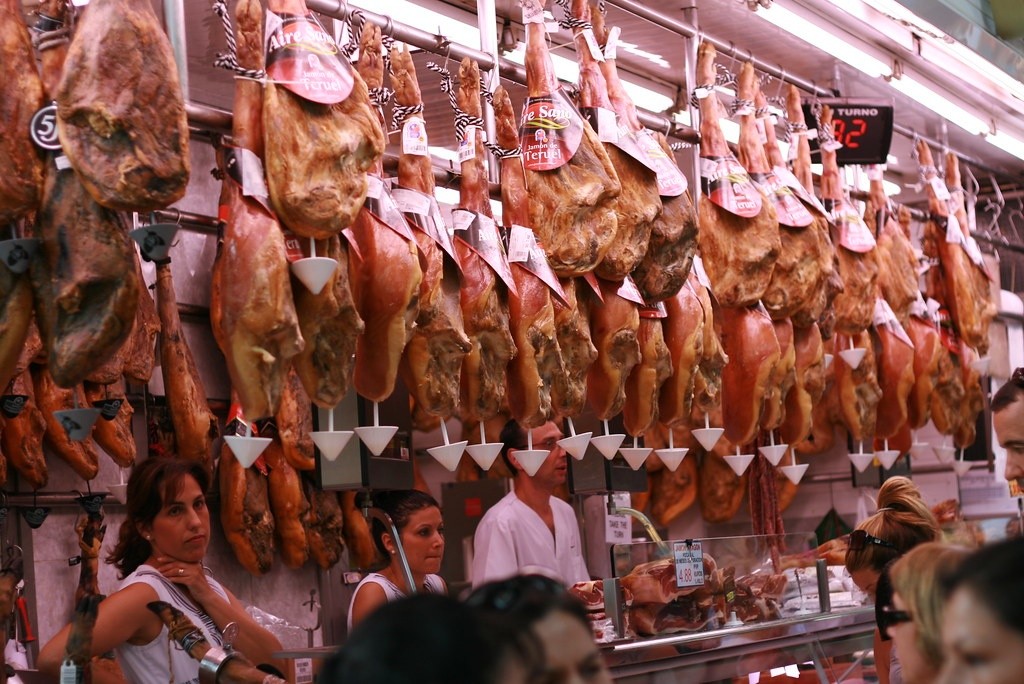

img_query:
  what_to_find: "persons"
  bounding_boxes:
[473,420,591,590]
[881,542,971,684]
[35,455,286,684]
[346,487,449,638]
[466,573,613,684]
[315,591,547,684]
[935,536,1024,684]
[990,368,1024,494]
[845,476,943,603]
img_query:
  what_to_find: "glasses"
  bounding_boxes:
[880,604,914,638]
[846,529,902,551]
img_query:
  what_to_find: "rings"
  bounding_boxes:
[179,568,183,576]
